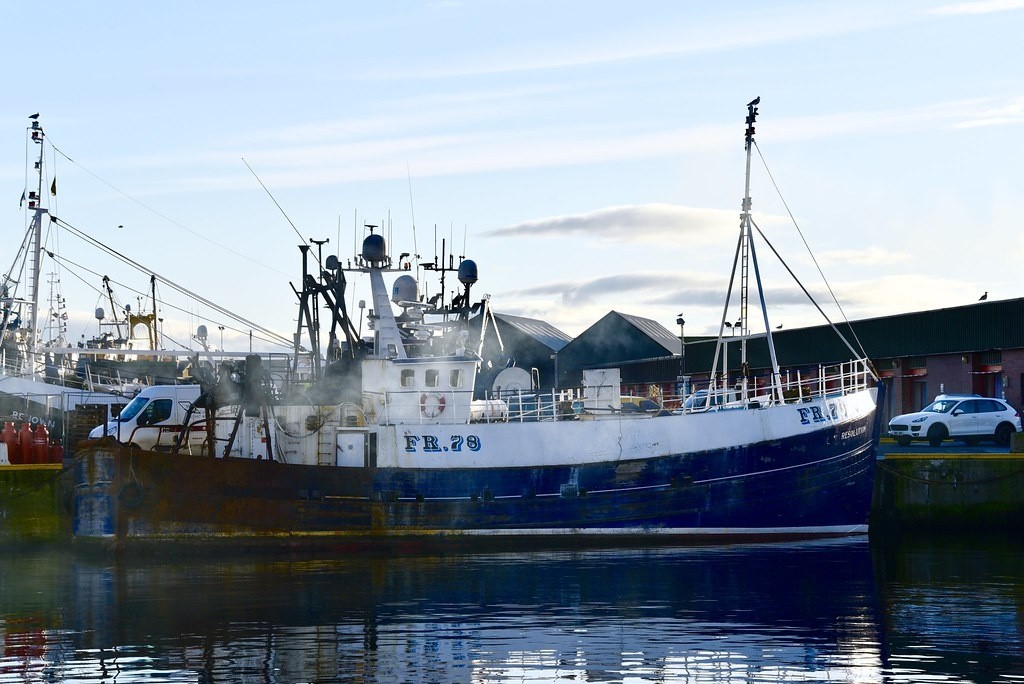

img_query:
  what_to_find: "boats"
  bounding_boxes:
[72,96,890,547]
[1,113,331,441]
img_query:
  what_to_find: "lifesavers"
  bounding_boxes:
[419,391,446,418]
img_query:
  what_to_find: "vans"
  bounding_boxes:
[672,389,737,414]
[88,384,212,451]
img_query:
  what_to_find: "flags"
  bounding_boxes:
[51,177,56,196]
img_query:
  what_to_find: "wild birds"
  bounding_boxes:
[747,96,762,108]
[738,317,741,321]
[978,291,989,301]
[775,323,783,331]
[28,112,40,119]
[677,313,683,317]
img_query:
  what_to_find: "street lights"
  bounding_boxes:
[676,314,686,383]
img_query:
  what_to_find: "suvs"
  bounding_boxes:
[888,397,1022,446]
